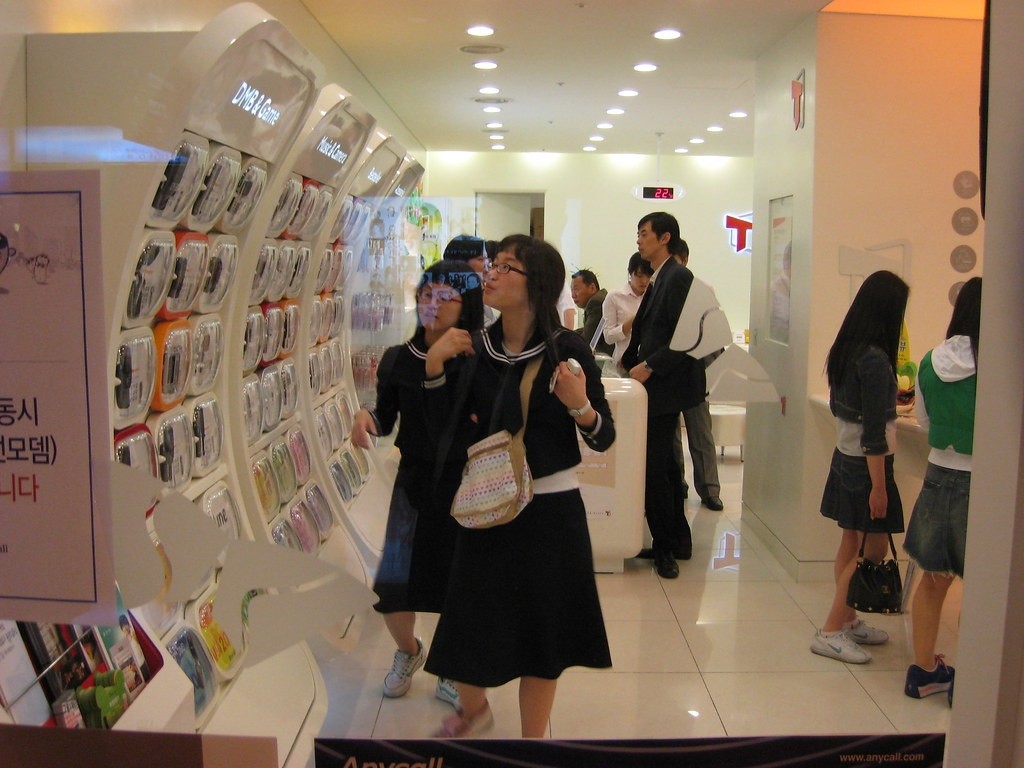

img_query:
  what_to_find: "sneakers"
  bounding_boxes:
[809,628,872,664]
[843,620,889,644]
[904,653,955,698]
[431,699,495,737]
[383,637,426,699]
[435,676,459,709]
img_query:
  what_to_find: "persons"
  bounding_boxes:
[614,213,708,578]
[904,278,983,708]
[443,235,726,510]
[351,261,484,704]
[119,615,150,683]
[424,234,614,738]
[771,240,791,344]
[810,270,910,664]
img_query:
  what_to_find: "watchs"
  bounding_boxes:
[568,400,590,417]
[643,360,653,372]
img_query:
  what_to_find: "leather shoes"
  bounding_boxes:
[634,545,691,560]
[654,549,679,579]
[701,497,723,511]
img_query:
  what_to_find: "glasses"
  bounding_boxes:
[417,288,462,305]
[484,262,527,277]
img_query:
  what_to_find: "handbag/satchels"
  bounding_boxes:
[845,558,903,614]
[451,429,535,529]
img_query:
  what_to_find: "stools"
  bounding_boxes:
[708,403,749,462]
